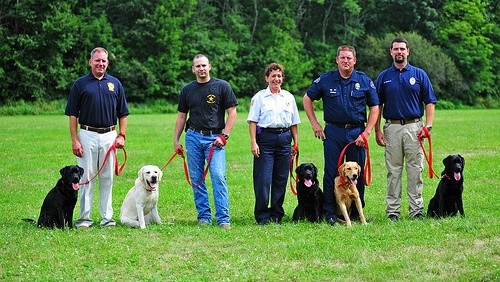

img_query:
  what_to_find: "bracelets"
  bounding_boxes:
[425,124,432,131]
[222,133,229,140]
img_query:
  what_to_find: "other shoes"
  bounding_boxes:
[416,213,426,220]
[78,226,88,232]
[258,222,264,225]
[221,223,230,230]
[352,216,360,222]
[388,215,398,222]
[199,221,209,225]
[326,216,336,227]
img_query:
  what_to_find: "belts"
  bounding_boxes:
[326,123,363,129]
[80,125,115,133]
[262,128,290,134]
[190,126,222,135]
[387,117,422,125]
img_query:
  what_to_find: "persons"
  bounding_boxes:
[376,38,437,221]
[303,46,380,224]
[174,54,238,227]
[247,62,301,225]
[65,47,129,228]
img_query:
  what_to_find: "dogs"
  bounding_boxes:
[20,164,85,231]
[119,163,163,229]
[291,162,324,224]
[425,153,467,220]
[333,160,368,228]
